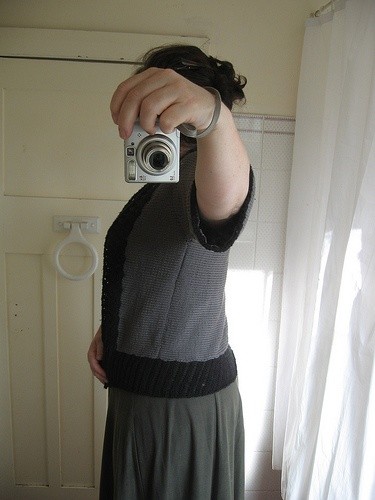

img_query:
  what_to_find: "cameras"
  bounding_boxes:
[123,116,181,183]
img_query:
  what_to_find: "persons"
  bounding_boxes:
[85,41,254,500]
